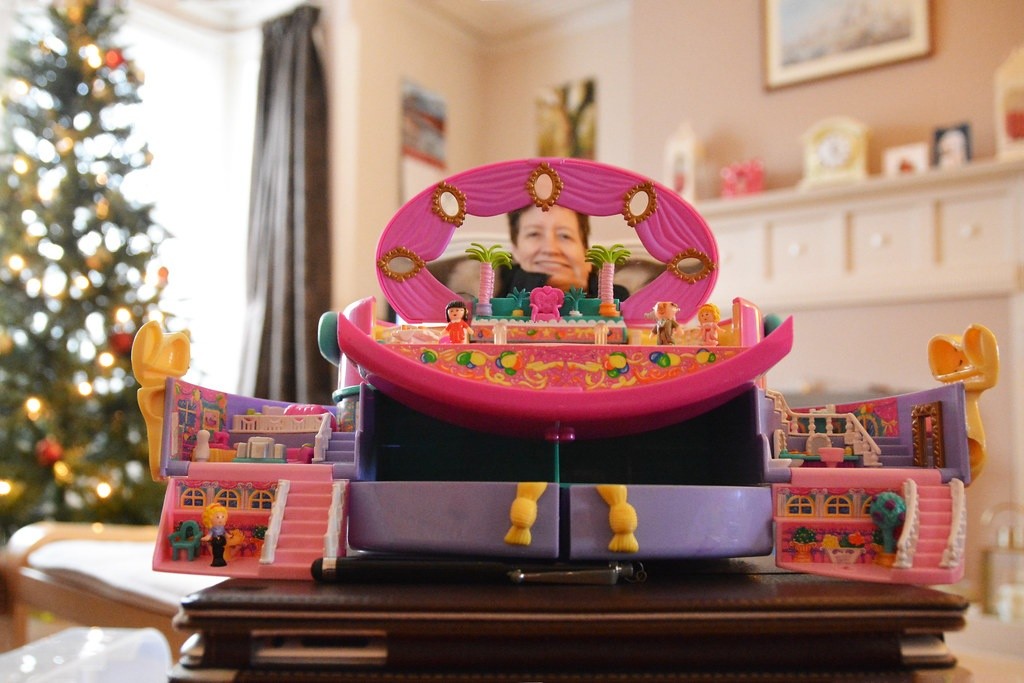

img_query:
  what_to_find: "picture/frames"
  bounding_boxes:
[761,1,936,91]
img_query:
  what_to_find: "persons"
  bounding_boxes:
[496,203,631,302]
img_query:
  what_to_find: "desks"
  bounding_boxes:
[3,521,239,664]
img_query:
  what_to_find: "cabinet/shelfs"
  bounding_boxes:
[680,153,1021,319]
